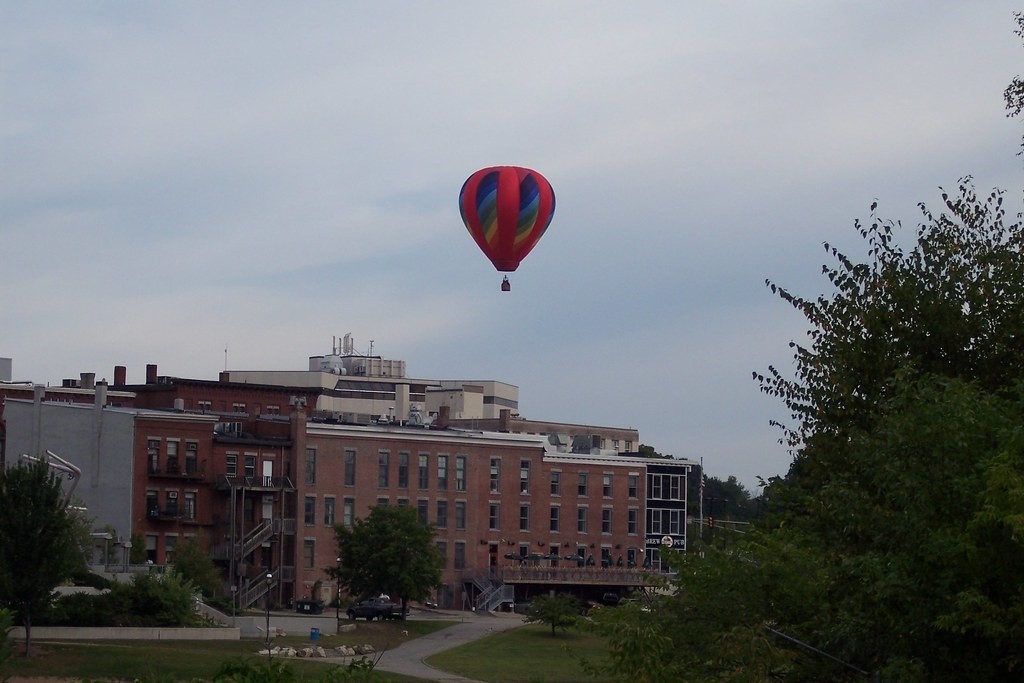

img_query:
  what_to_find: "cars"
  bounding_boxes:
[578,600,604,616]
[347,598,409,621]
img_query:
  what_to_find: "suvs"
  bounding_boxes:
[618,598,656,613]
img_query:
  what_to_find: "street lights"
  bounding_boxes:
[266,574,272,643]
[336,555,340,617]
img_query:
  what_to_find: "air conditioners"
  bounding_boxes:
[230,422,242,433]
[169,492,177,499]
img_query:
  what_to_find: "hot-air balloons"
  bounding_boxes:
[458,166,556,292]
